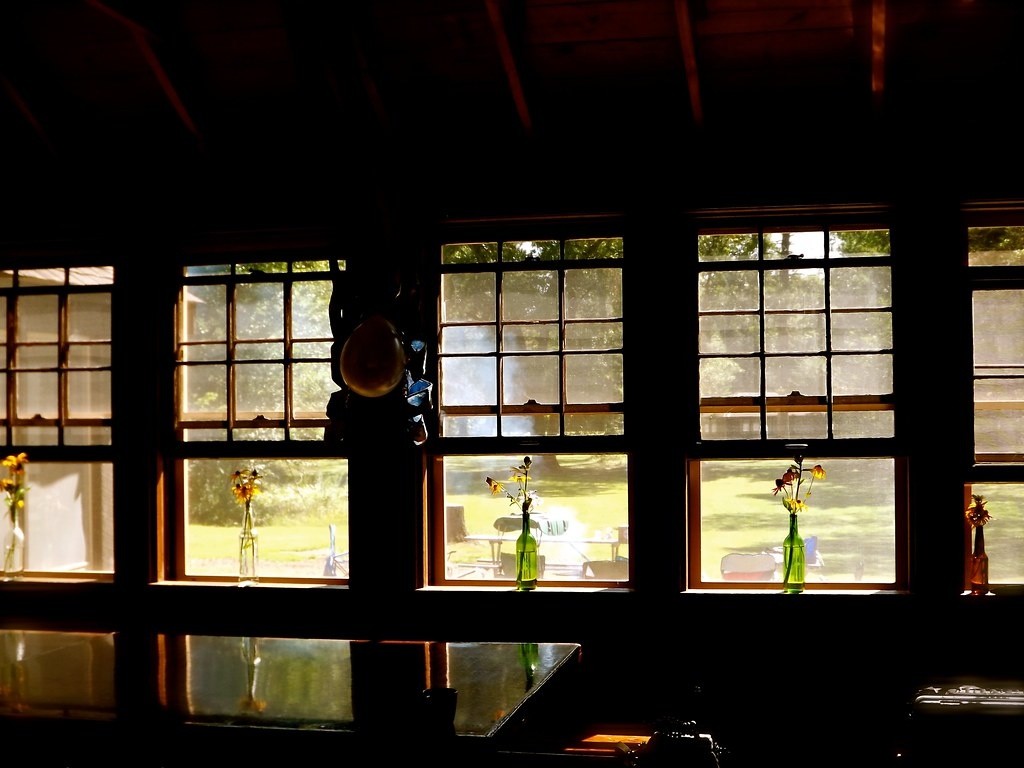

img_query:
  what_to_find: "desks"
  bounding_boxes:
[0,628,584,768]
[464,534,620,575]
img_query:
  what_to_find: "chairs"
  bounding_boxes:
[720,536,825,585]
[613,526,627,562]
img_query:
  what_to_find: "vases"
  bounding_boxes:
[969,528,988,591]
[783,515,804,593]
[516,512,538,590]
[239,508,258,584]
[3,507,24,575]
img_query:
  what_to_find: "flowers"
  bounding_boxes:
[772,454,824,514]
[966,493,991,527]
[483,456,534,512]
[0,454,30,511]
[227,467,260,504]
